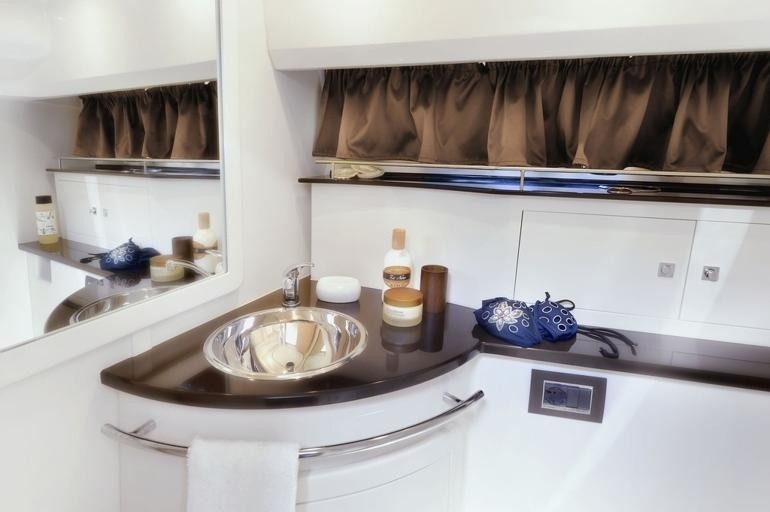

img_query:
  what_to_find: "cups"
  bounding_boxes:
[420,264,448,314]
[172,236,193,278]
[420,314,445,351]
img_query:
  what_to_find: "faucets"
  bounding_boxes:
[277,259,313,307]
[166,259,208,278]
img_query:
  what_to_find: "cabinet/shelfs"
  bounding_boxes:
[510,208,770,331]
[44,181,159,252]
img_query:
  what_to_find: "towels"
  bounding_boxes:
[189,437,299,511]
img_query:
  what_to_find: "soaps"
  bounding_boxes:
[316,276,362,304]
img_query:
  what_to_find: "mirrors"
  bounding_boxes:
[1,0,246,392]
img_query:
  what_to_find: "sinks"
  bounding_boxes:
[203,305,369,382]
[71,283,187,322]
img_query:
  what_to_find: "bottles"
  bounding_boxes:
[194,213,221,275]
[381,227,412,303]
[34,195,60,244]
